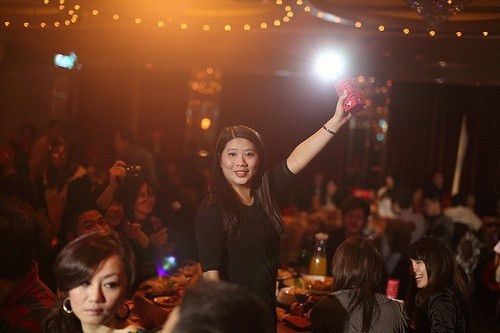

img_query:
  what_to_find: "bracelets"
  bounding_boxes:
[323,124,336,135]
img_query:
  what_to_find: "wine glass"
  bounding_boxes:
[294,282,312,315]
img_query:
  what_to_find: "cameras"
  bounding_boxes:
[124,163,145,178]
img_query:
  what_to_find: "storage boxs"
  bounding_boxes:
[386,279,400,299]
[335,76,366,115]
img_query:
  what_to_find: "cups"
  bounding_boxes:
[386,279,399,299]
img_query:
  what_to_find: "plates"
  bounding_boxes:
[283,314,312,331]
[154,296,183,306]
[283,274,334,295]
[277,268,292,280]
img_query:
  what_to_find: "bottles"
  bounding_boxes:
[309,235,327,276]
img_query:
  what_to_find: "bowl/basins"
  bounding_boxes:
[278,286,313,301]
[173,283,187,297]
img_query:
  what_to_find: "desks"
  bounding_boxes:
[129,276,320,333]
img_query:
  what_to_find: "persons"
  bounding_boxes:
[0,124,500,333]
[196,91,354,333]
